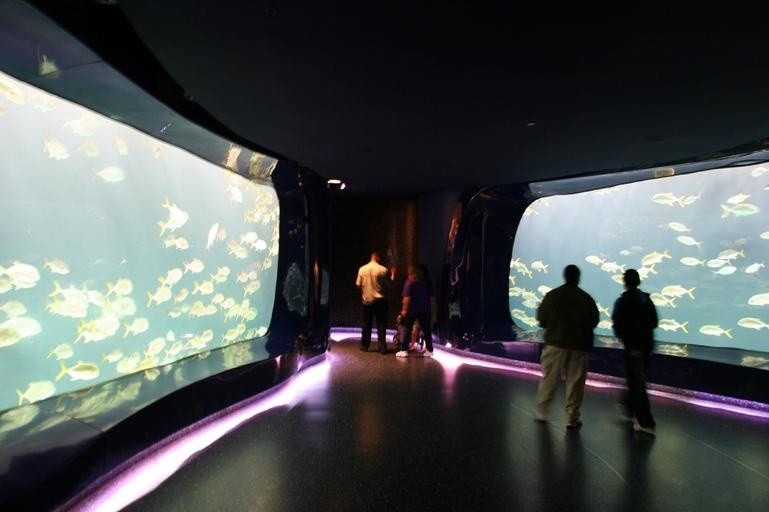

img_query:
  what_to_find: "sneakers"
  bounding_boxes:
[633,423,654,434]
[424,350,434,356]
[614,402,633,424]
[360,347,368,352]
[567,418,583,428]
[396,350,409,358]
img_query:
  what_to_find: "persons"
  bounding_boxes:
[611,270,660,436]
[534,264,600,428]
[394,265,434,357]
[355,252,396,352]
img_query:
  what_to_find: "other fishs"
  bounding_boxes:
[0,258,278,407]
